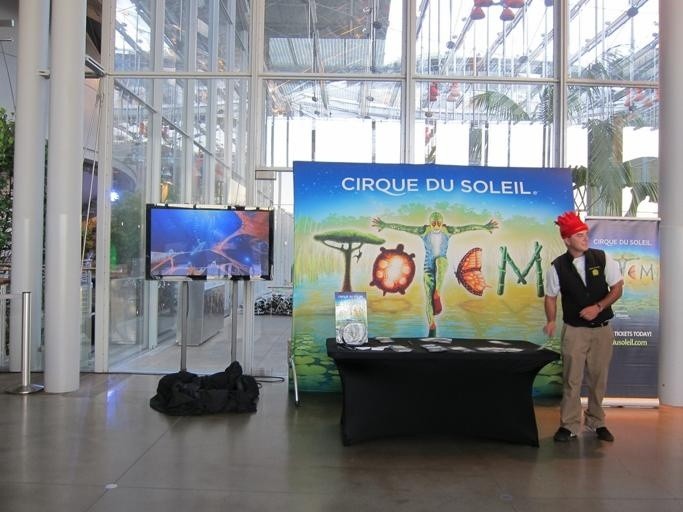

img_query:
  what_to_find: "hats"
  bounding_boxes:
[553,210,589,239]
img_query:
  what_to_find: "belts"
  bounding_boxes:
[584,321,610,328]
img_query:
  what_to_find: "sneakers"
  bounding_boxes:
[553,427,577,442]
[584,423,614,443]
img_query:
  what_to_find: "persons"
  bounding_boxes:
[372,209,497,337]
[540,210,624,444]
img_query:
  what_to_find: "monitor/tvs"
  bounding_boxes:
[146,202,275,281]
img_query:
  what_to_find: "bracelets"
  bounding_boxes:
[596,302,603,313]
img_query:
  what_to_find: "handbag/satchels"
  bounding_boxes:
[149,361,260,417]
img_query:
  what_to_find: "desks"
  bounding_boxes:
[325,338,559,448]
